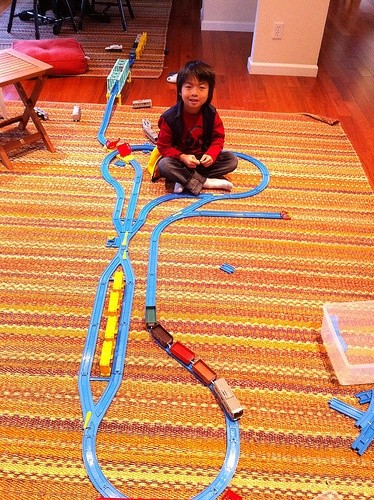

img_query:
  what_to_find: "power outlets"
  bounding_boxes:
[272,23,284,39]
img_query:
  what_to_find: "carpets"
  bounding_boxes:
[0,0,172,79]
[0,100,374,500]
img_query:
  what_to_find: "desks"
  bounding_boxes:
[0,51,57,171]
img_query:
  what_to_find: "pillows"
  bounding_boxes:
[12,37,90,79]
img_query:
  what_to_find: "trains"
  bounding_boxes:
[144,304,245,422]
[142,119,158,144]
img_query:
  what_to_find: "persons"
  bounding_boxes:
[157,59,238,194]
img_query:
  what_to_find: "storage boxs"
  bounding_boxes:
[320,300,374,385]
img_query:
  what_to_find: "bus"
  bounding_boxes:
[72,105,81,122]
[34,107,47,120]
[133,99,153,109]
